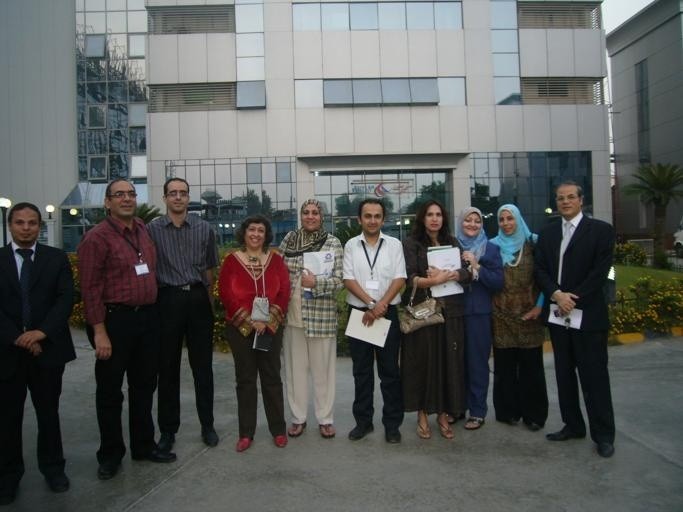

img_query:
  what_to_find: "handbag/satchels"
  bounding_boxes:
[397,276,445,334]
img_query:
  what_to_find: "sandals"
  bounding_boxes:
[464,417,483,430]
[448,412,464,423]
[320,424,336,439]
[289,422,307,437]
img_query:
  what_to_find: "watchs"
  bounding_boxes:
[367,299,378,310]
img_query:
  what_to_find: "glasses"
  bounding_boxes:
[165,192,188,197]
[106,191,137,198]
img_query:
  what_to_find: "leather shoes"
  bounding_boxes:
[349,424,373,440]
[131,449,176,462]
[546,428,586,440]
[598,442,614,458]
[202,428,217,446]
[274,435,287,448]
[157,434,174,452]
[236,439,251,451]
[386,428,401,443]
[98,464,115,480]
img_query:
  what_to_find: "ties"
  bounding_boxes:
[15,249,35,329]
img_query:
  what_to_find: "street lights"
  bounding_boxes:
[45,203,55,245]
[544,207,552,226]
[69,207,88,236]
[0,197,12,247]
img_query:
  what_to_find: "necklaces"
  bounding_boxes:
[242,248,267,262]
[506,247,526,269]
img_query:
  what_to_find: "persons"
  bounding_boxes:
[441,207,505,428]
[0,202,77,504]
[149,179,219,451]
[535,182,616,456]
[485,203,549,432]
[399,199,471,439]
[273,200,344,436]
[222,214,291,450]
[77,180,176,479]
[341,201,408,444]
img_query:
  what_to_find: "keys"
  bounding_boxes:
[553,308,572,330]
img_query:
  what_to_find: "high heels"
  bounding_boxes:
[417,420,430,439]
[439,420,454,439]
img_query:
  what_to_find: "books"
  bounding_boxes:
[426,244,464,297]
[253,329,272,353]
[346,307,392,347]
[545,302,583,330]
[302,252,336,299]
